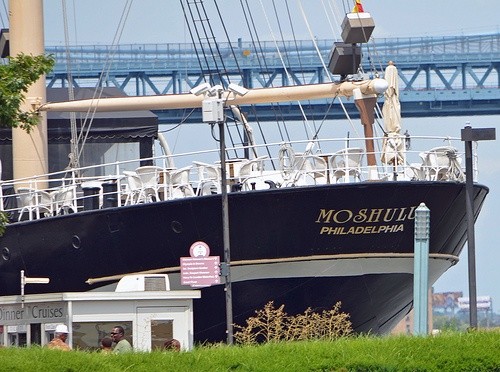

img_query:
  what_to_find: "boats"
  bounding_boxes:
[0,2,490,348]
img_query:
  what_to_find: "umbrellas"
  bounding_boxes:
[381,60,404,182]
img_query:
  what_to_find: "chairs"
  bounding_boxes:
[330,147,365,185]
[15,187,54,222]
[409,146,462,182]
[123,154,268,208]
[50,185,78,215]
[296,153,328,186]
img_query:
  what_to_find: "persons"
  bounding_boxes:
[111,326,131,353]
[100,337,113,354]
[168,339,180,353]
[46,324,69,350]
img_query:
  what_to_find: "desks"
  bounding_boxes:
[214,159,243,193]
[41,188,59,218]
[316,153,337,182]
[158,168,179,201]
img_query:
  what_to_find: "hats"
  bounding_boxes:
[55,324,70,334]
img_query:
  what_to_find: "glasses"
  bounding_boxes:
[110,332,119,335]
